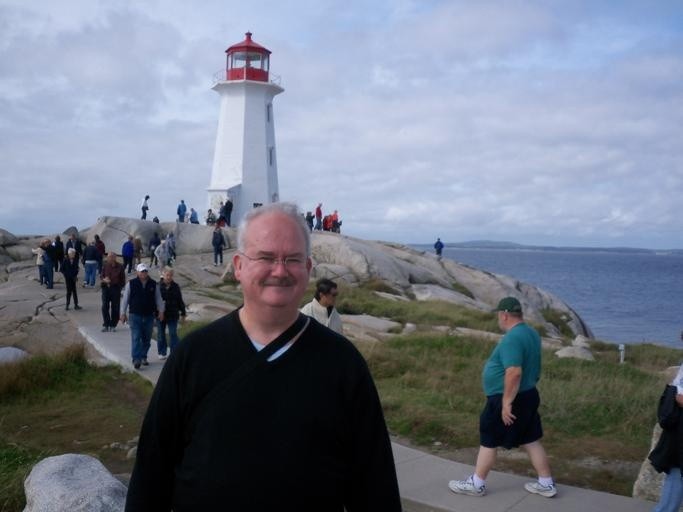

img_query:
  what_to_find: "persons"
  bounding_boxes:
[299,280,343,336]
[119,262,166,368]
[154,266,186,360]
[447,297,558,498]
[60,248,82,310]
[301,203,341,234]
[435,238,444,256]
[654,332,683,512]
[97,252,125,331]
[121,201,403,510]
[31,195,233,289]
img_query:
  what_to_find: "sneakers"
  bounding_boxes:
[110,327,116,332]
[142,358,148,365]
[522,480,558,497]
[100,326,108,332]
[133,359,140,368]
[448,476,486,497]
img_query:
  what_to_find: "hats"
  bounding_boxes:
[492,297,523,315]
[135,263,150,272]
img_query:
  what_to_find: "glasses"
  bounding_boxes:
[139,270,148,273]
[329,290,340,297]
[240,250,311,272]
